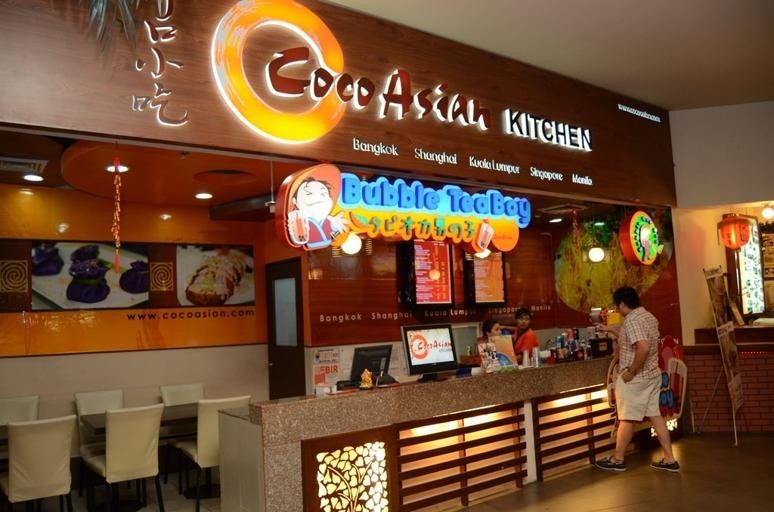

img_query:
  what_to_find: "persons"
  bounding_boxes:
[588,285,681,473]
[474,320,501,368]
[509,308,539,366]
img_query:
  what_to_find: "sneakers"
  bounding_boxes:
[596,456,626,471]
[651,458,680,472]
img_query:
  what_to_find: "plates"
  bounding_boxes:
[176,248,255,306]
[32,243,150,310]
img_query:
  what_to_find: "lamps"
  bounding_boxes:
[585,214,608,263]
[716,213,752,252]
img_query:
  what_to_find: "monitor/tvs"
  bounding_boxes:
[351,344,395,385]
[401,323,458,385]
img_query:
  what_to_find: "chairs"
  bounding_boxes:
[0,382,251,512]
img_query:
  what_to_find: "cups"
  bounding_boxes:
[523,346,542,369]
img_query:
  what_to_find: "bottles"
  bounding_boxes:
[573,327,580,340]
[562,329,572,346]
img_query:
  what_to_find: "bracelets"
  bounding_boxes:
[626,366,638,376]
